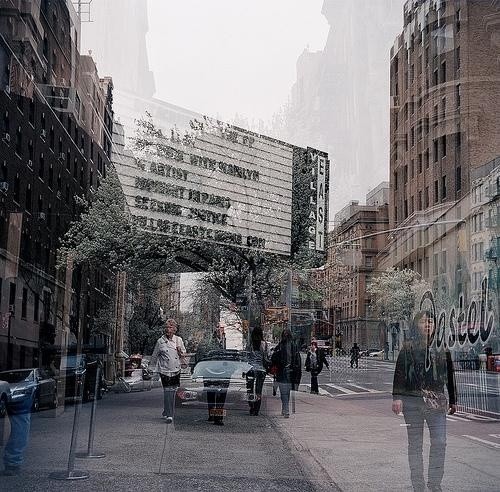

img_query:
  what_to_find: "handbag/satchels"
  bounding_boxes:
[273,373,278,396]
[422,388,448,412]
[175,335,189,369]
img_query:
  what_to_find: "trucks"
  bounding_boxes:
[488,356,500,372]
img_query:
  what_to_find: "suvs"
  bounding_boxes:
[53,354,103,401]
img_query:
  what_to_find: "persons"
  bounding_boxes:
[191,317,235,427]
[349,341,361,369]
[303,339,330,393]
[267,329,302,419]
[147,317,187,424]
[241,324,273,417]
[0,273,42,476]
[391,309,460,492]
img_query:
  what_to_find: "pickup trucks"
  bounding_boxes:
[308,340,333,356]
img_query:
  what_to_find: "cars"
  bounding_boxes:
[174,358,267,433]
[1,368,58,413]
[458,354,479,369]
[112,369,152,393]
[359,348,384,358]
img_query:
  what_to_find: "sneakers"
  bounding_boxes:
[427,482,442,491]
[163,416,172,423]
[207,416,224,426]
[282,410,290,418]
[249,410,260,416]
[5,465,18,476]
